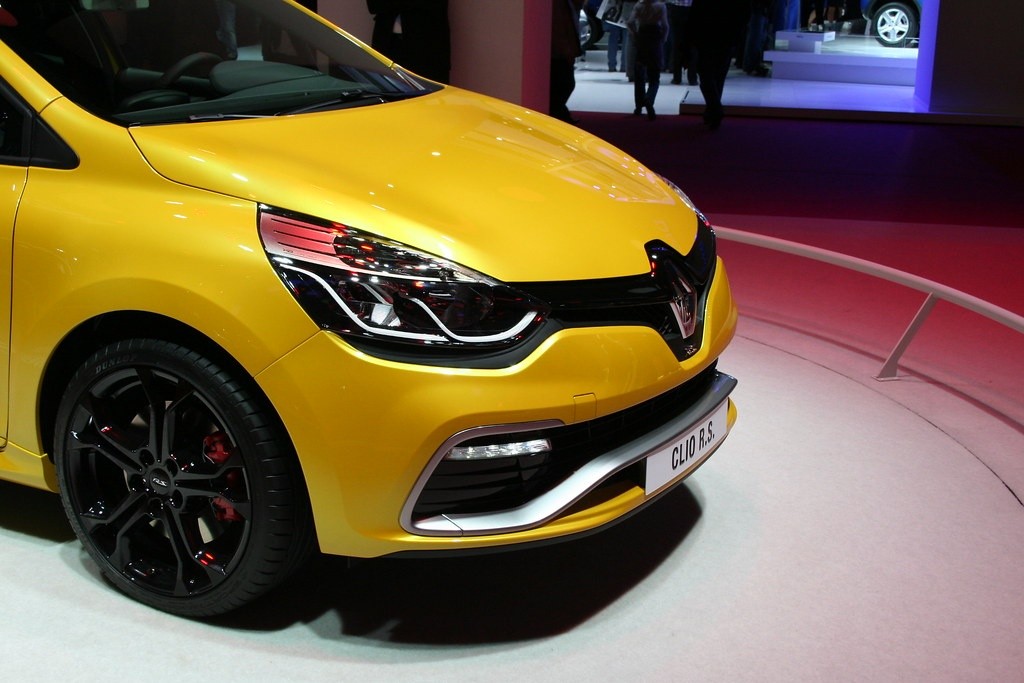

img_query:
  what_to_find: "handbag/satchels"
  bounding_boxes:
[595,0,635,28]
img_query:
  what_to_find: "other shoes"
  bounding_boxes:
[736,62,742,68]
[620,69,625,72]
[671,79,681,84]
[645,100,656,120]
[689,80,698,85]
[634,110,641,114]
[609,68,616,71]
[703,109,713,121]
[710,110,723,131]
[748,68,769,76]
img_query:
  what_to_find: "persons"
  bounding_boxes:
[365,0,452,85]
[258,0,318,72]
[550,0,771,125]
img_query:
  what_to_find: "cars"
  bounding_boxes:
[855,0,924,47]
[0,1,743,617]
